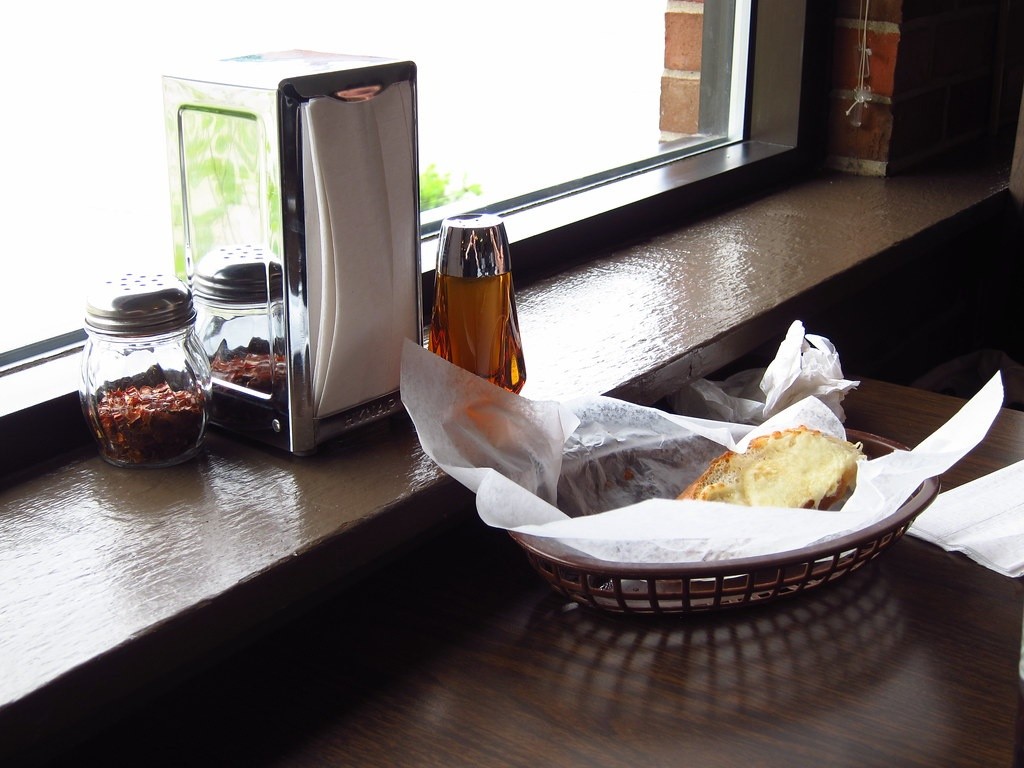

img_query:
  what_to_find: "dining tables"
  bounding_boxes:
[191,362,1024,768]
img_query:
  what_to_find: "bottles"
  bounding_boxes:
[427,213,526,397]
[197,242,285,390]
[80,270,213,467]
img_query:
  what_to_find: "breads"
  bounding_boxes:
[665,425,865,511]
